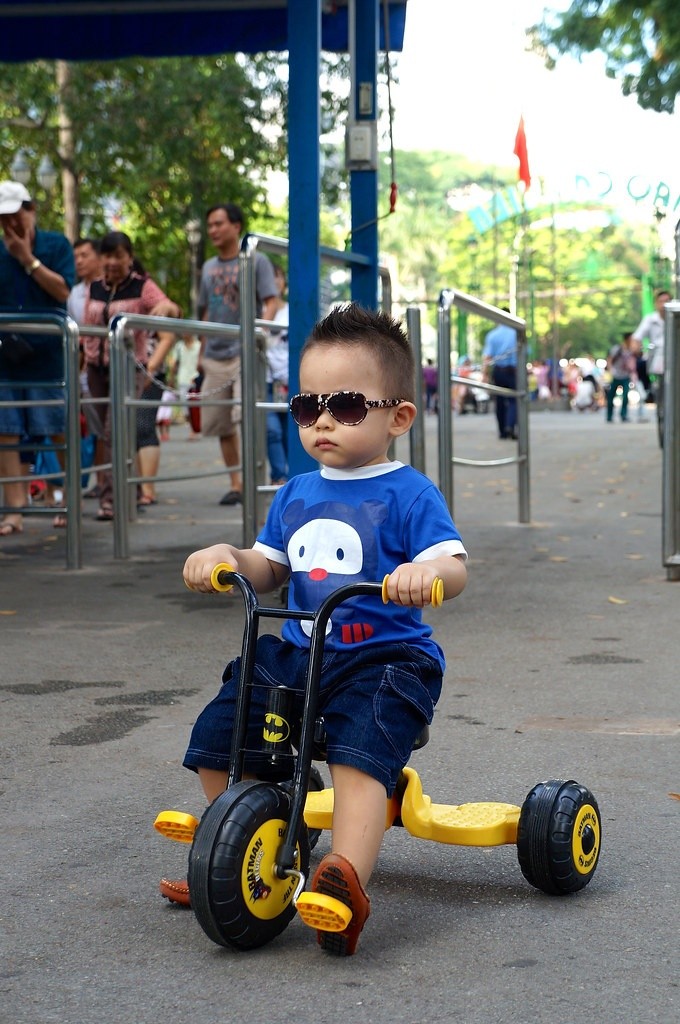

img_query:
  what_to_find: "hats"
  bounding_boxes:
[0,181,33,215]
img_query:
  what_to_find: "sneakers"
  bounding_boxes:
[311,852,371,957]
[160,877,191,906]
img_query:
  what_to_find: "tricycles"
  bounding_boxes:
[149,562,606,954]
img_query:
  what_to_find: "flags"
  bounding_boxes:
[514,119,530,189]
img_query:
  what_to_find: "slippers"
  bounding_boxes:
[138,495,158,506]
[52,513,68,528]
[1,512,25,535]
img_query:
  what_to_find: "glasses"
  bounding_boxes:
[289,391,405,428]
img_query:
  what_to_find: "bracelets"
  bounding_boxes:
[26,260,41,275]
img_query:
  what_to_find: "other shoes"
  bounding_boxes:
[220,491,243,506]
[506,429,519,441]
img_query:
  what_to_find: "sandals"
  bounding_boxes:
[96,504,115,520]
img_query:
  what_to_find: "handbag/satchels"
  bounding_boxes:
[188,375,201,431]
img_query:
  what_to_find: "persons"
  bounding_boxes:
[159,301,469,957]
[629,291,672,374]
[0,180,290,536]
[420,353,473,414]
[480,307,532,440]
[527,332,653,423]
